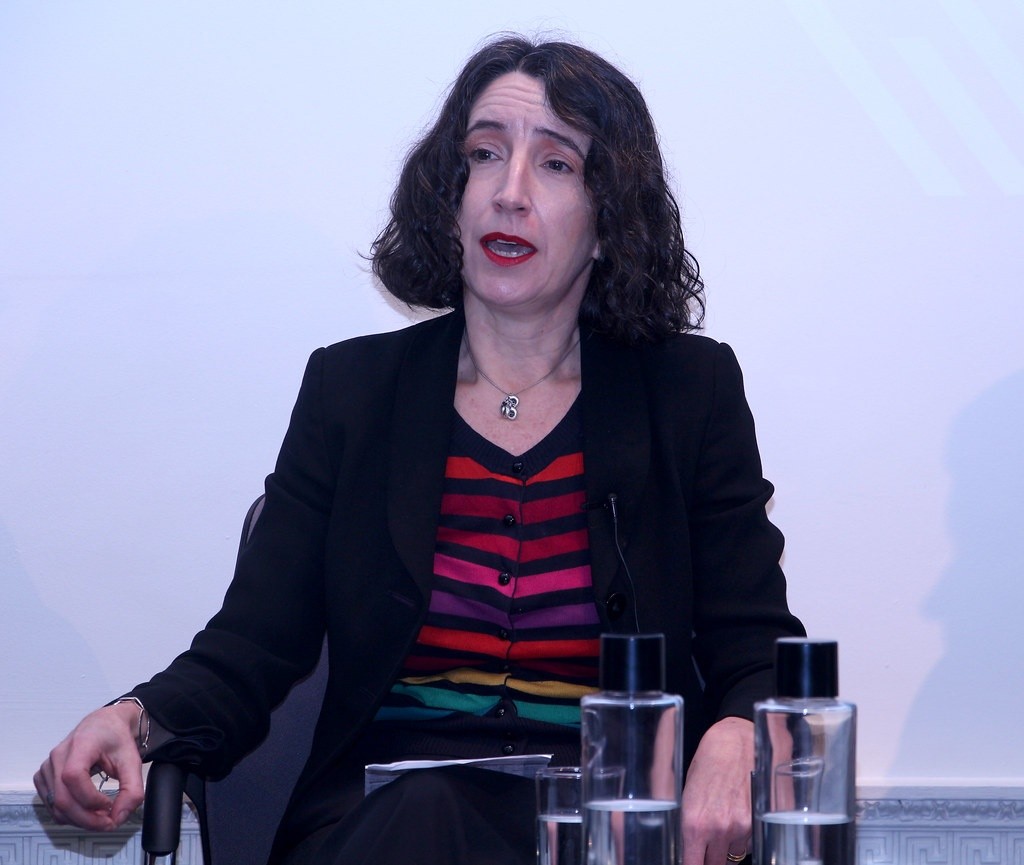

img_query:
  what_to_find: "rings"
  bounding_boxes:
[727,849,747,863]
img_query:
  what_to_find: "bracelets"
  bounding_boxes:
[94,695,151,792]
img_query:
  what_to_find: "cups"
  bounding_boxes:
[533,764,630,865]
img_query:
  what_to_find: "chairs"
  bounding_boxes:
[140,493,329,863]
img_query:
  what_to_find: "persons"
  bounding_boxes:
[29,37,814,865]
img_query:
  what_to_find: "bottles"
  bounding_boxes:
[580,631,685,865]
[753,635,861,865]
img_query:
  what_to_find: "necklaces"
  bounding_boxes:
[461,326,580,422]
